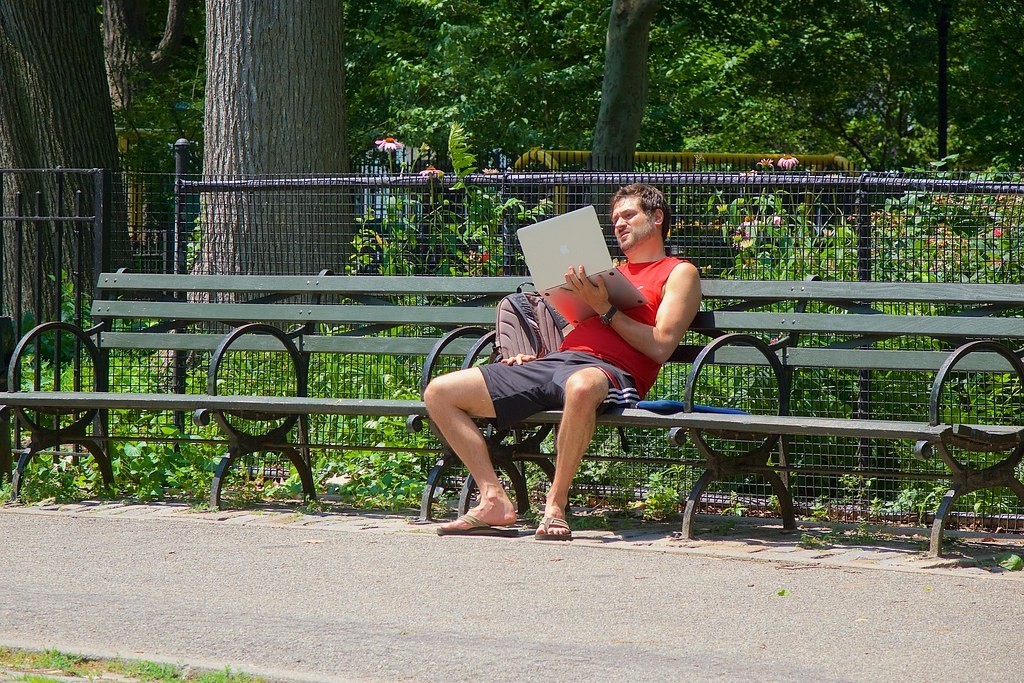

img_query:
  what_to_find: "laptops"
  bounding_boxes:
[517,206,649,328]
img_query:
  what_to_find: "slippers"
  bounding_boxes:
[437,515,518,537]
[535,517,572,540]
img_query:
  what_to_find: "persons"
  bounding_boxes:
[424,183,702,541]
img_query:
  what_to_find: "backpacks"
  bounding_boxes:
[496,282,564,359]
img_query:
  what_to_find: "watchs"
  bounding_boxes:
[599,304,618,327]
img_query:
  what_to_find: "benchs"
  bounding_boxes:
[1,267,1024,558]
